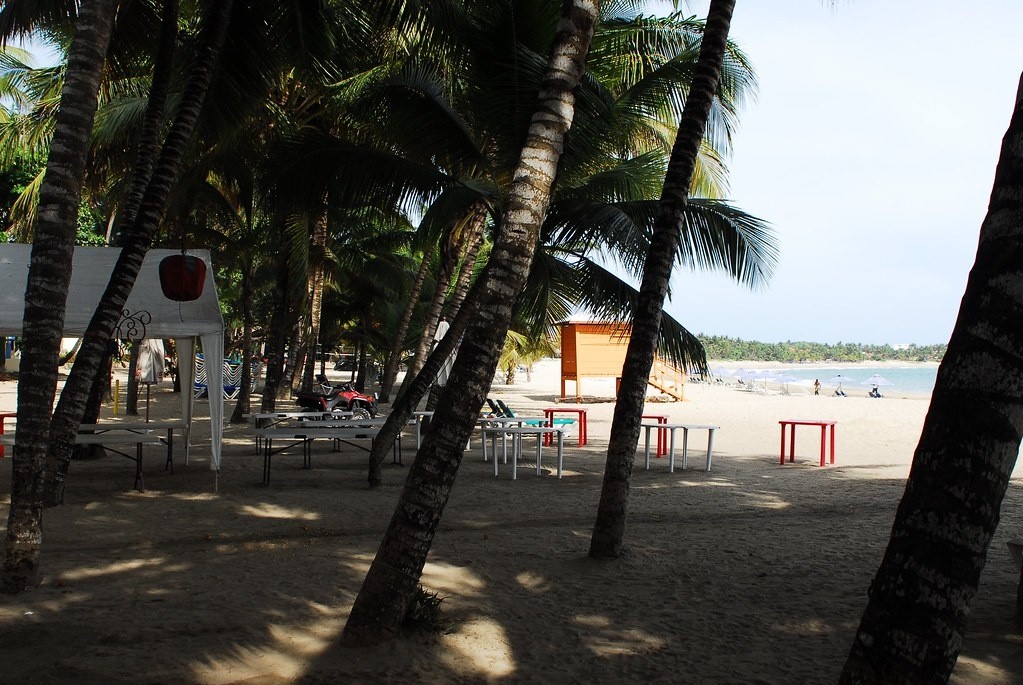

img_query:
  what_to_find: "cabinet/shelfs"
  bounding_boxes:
[488,416,544,456]
[484,426,564,481]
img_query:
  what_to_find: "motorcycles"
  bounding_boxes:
[294,381,378,428]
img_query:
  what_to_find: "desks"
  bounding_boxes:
[777,419,838,468]
[67,420,187,494]
[641,414,670,458]
[253,411,406,486]
[640,422,723,473]
[543,406,589,448]
